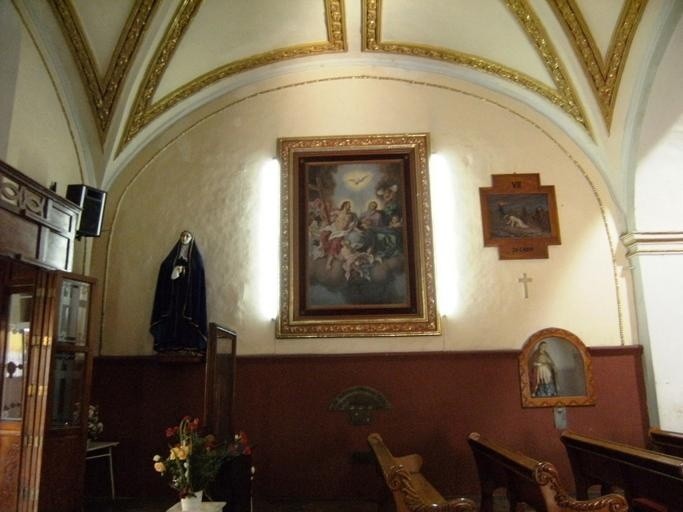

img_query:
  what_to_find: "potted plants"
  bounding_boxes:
[272,132,443,339]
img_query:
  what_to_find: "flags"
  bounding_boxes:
[180,487,204,512]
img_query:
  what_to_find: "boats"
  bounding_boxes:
[67,183,107,237]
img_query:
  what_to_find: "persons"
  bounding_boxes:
[364,213,402,266]
[377,179,402,214]
[148,228,209,353]
[533,205,549,227]
[314,198,356,272]
[495,199,514,218]
[521,205,531,224]
[350,199,386,235]
[526,339,560,396]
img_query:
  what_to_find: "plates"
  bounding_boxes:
[1,156,102,511]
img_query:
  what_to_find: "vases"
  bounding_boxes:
[150,412,254,500]
